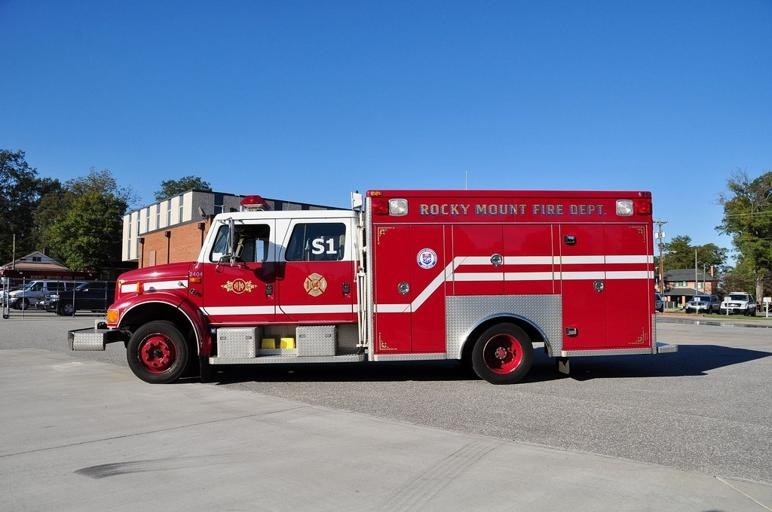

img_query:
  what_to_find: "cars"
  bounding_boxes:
[0,279,114,316]
[653,293,665,314]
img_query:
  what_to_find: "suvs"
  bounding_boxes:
[720,291,756,319]
[684,294,724,317]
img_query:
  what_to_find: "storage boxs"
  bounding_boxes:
[216,326,262,358]
[296,325,336,357]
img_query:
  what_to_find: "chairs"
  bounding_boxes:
[314,235,339,260]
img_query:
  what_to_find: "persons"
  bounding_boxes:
[229,231,253,262]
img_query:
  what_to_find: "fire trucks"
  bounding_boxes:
[66,188,678,386]
